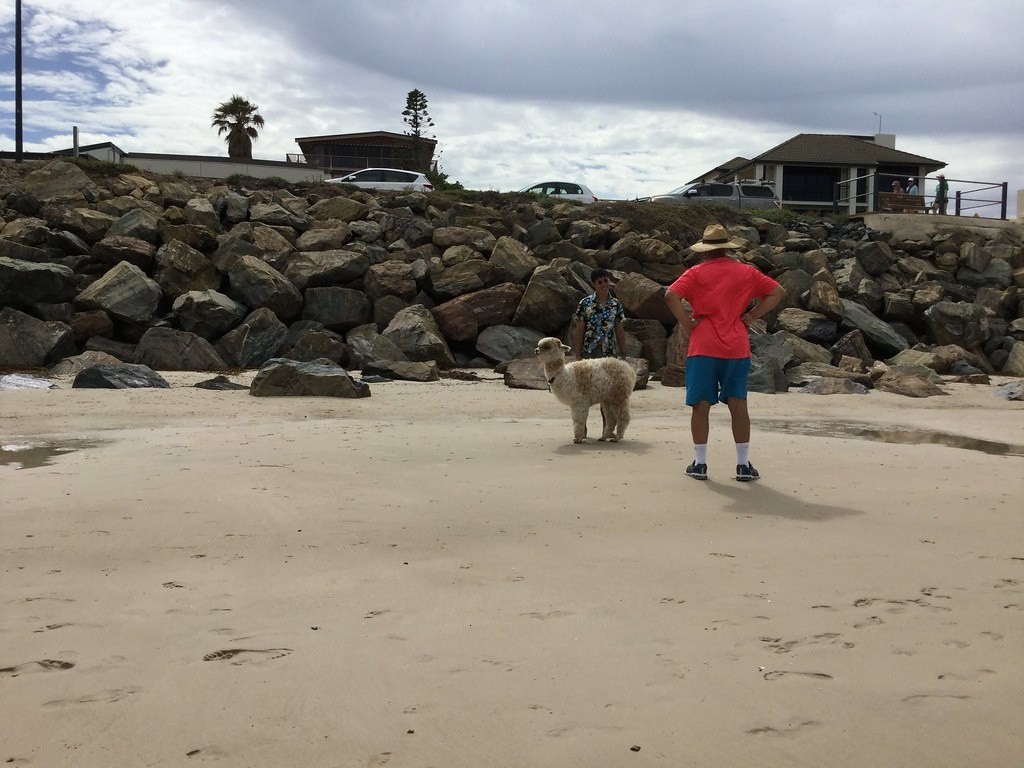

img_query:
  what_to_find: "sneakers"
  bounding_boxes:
[684,460,707,480]
[736,462,760,482]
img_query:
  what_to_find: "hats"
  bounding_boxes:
[689,224,742,252]
[937,175,944,178]
[909,178,913,180]
[892,181,899,185]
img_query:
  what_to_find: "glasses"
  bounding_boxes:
[595,279,608,285]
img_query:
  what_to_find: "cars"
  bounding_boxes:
[325,167,436,193]
[518,181,599,206]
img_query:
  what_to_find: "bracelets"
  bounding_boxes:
[621,352,627,355]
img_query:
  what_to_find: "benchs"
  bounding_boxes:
[878,192,933,214]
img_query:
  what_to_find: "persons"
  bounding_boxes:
[906,177,918,195]
[932,174,949,215]
[892,181,904,194]
[664,223,785,482]
[575,268,627,440]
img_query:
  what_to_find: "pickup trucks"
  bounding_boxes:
[649,177,782,213]
